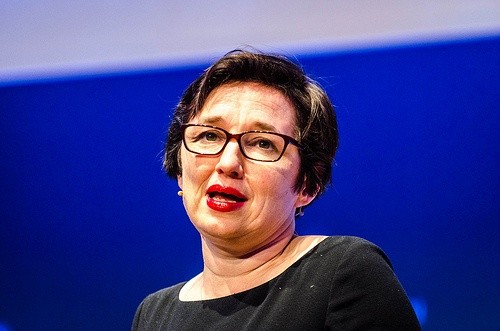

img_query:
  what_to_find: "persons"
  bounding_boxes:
[129,48,422,331]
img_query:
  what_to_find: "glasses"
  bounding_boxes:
[175,117,304,163]
